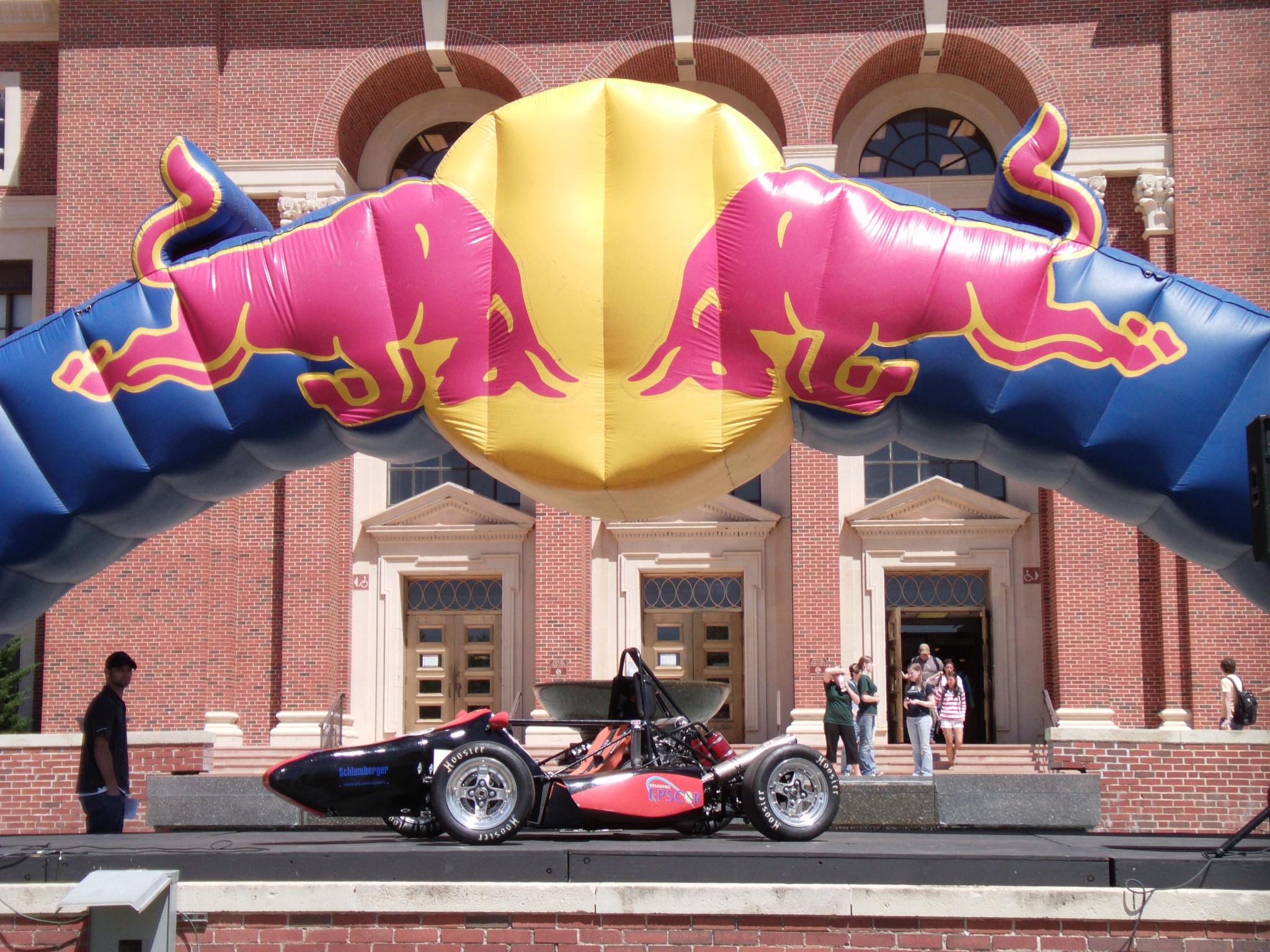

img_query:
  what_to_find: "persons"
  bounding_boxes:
[74,651,138,835]
[934,671,966,771]
[900,643,945,744]
[925,659,963,745]
[823,656,884,777]
[1218,658,1258,730]
[903,663,936,776]
[1261,687,1270,696]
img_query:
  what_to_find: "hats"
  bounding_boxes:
[919,643,930,655]
[106,651,137,670]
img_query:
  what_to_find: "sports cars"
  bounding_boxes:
[262,648,841,846]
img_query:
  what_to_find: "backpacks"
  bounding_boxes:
[923,682,936,727]
[1227,676,1258,726]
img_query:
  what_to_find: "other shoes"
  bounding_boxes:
[876,771,883,776]
[949,764,954,771]
[952,746,957,761]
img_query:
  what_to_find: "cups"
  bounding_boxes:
[838,675,847,689]
